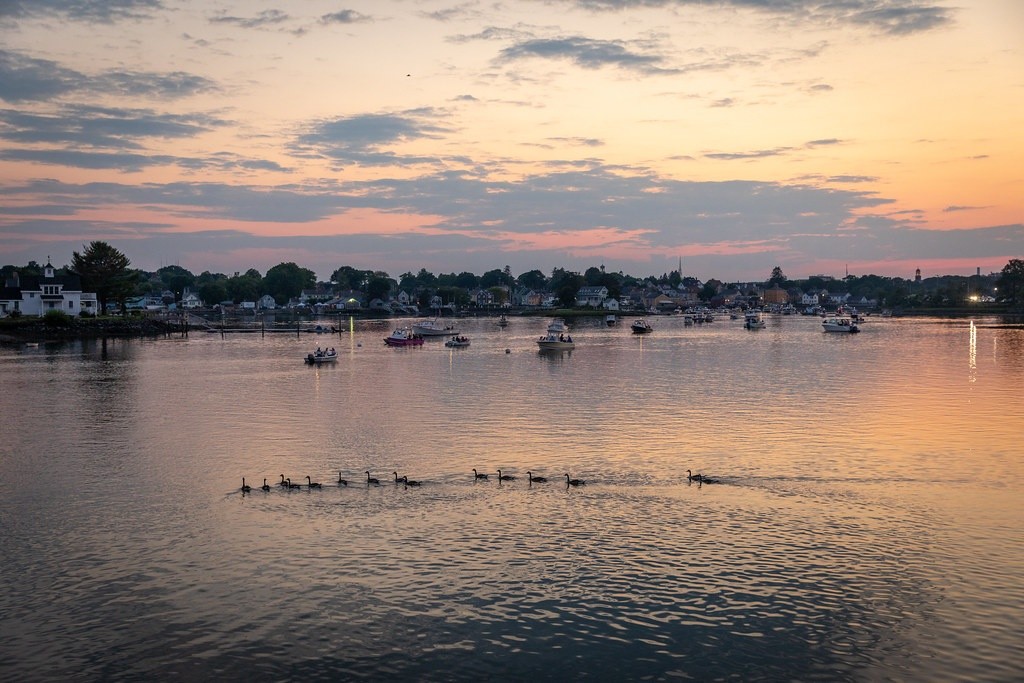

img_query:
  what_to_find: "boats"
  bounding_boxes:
[499,315,509,326]
[743,308,766,329]
[764,304,894,323]
[304,352,338,363]
[821,316,860,333]
[630,319,653,333]
[536,319,576,349]
[447,340,471,345]
[606,315,616,325]
[647,305,742,327]
[383,328,425,345]
[411,318,461,335]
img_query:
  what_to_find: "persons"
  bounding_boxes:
[325,347,329,355]
[844,321,848,326]
[859,317,861,319]
[851,320,855,327]
[559,333,564,342]
[848,321,851,326]
[823,320,828,323]
[829,319,832,322]
[546,336,548,341]
[415,332,419,337]
[453,336,456,342]
[563,338,567,343]
[329,348,335,356]
[543,336,546,341]
[567,335,572,343]
[451,325,454,329]
[409,332,412,339]
[841,320,844,326]
[832,319,835,322]
[856,318,858,320]
[419,333,424,340]
[553,335,556,338]
[314,346,323,357]
[549,335,554,341]
[413,333,419,340]
[456,336,461,343]
[847,320,849,323]
[462,336,465,342]
[332,349,336,355]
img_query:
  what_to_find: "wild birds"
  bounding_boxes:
[496,469,515,481]
[685,470,720,484]
[240,477,251,494]
[338,472,347,486]
[280,474,300,489]
[365,471,380,483]
[526,471,548,483]
[262,478,270,492]
[564,473,585,486]
[392,471,422,486]
[472,468,488,479]
[305,475,322,489]
